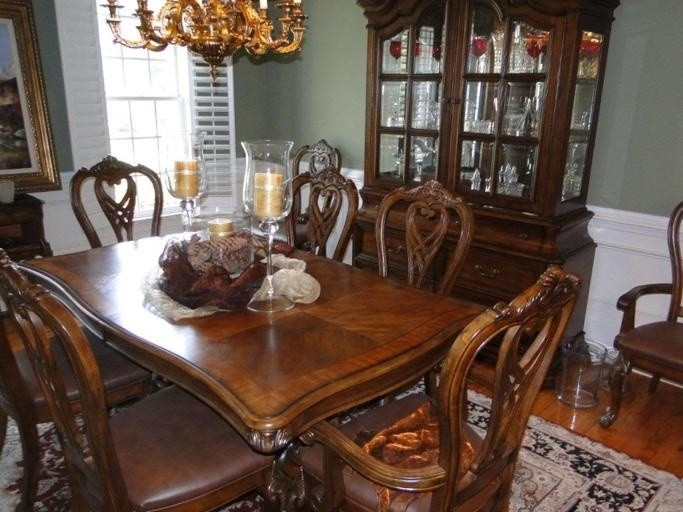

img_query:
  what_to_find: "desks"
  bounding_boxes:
[17,227,529,512]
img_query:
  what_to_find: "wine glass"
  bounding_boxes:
[388,30,601,76]
[153,129,207,239]
[240,138,297,313]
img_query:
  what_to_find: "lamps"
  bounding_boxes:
[99,0,313,81]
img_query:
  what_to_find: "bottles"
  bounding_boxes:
[470,157,582,199]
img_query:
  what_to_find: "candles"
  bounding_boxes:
[173,161,202,196]
[248,169,291,220]
[207,217,235,241]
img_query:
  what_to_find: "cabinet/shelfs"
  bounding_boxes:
[0,193,55,263]
[356,0,623,214]
[440,206,596,388]
[346,200,437,296]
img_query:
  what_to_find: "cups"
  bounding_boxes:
[554,335,634,410]
[465,81,589,138]
[0,180,16,204]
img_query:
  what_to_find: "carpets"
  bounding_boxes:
[0,378,682,512]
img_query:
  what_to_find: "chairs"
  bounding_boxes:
[283,166,362,263]
[373,176,476,397]
[252,137,341,251]
[597,202,681,428]
[0,315,152,512]
[67,153,164,249]
[0,247,272,512]
[303,264,580,512]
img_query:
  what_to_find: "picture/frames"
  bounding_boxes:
[0,0,64,197]
